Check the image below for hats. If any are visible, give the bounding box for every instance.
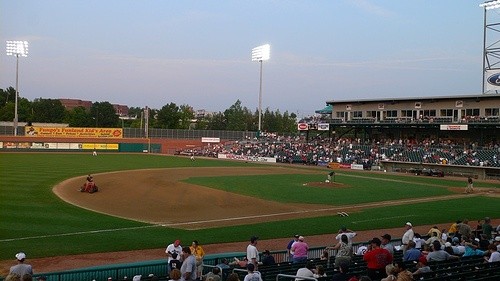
[294,234,300,238]
[16,252,26,260]
[250,236,258,241]
[298,236,304,241]
[452,237,459,242]
[406,222,412,227]
[371,238,381,246]
[263,249,270,252]
[484,217,489,221]
[381,234,391,240]
[419,256,427,264]
[174,240,180,246]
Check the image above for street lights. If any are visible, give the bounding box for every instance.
[249,41,272,130]
[4,38,30,126]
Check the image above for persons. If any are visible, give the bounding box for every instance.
[86,113,500,281]
[4,252,34,281]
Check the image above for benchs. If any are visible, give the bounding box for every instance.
[230,145,500,281]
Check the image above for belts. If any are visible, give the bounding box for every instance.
[402,244,408,245]
